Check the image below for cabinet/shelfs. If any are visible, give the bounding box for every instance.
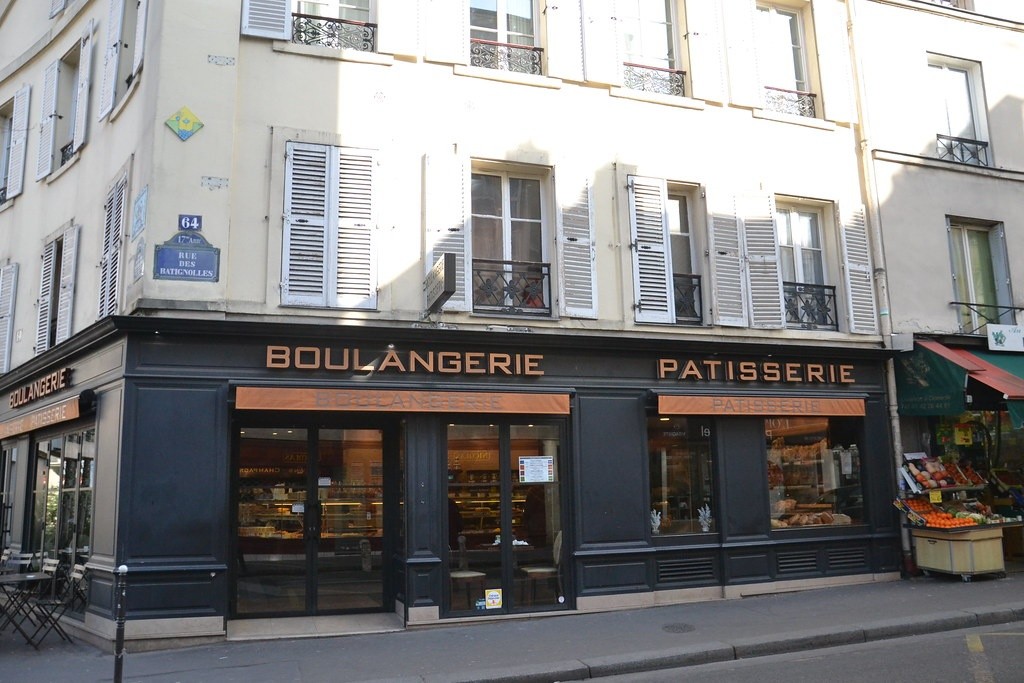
[770,459,825,489]
[239,483,546,553]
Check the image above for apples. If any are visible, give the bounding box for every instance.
[944,463,967,484]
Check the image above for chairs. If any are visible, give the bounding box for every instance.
[0,548,87,648]
[522,529,563,606]
[449,536,486,608]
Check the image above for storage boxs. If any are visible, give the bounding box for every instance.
[893,457,1024,526]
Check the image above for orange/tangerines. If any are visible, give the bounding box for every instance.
[902,499,977,528]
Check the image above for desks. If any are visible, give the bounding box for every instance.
[907,522,1024,581]
[0,572,53,651]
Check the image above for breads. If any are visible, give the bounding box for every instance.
[767,435,822,484]
[770,512,834,527]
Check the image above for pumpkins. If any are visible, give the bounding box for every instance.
[908,462,955,488]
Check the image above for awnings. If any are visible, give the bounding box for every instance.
[913,340,1024,399]
[896,350,966,417]
